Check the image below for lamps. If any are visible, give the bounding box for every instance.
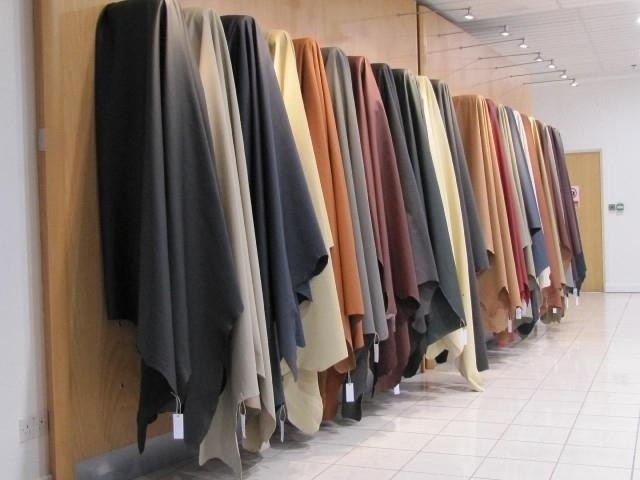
[478,51,545,62]
[460,37,528,49]
[398,6,475,20]
[438,24,510,36]
[495,58,555,69]
[523,78,580,87]
[510,69,568,79]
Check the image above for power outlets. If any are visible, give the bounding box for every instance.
[608,204,615,210]
